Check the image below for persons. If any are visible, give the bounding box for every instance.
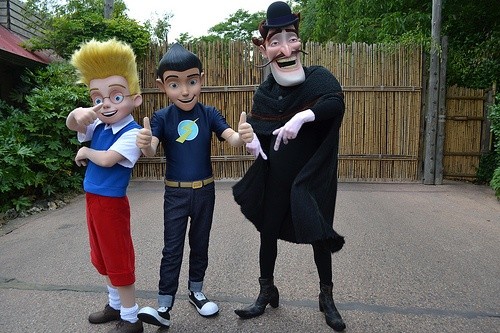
[136,43,253,328]
[66,39,142,333]
[232,1,347,331]
